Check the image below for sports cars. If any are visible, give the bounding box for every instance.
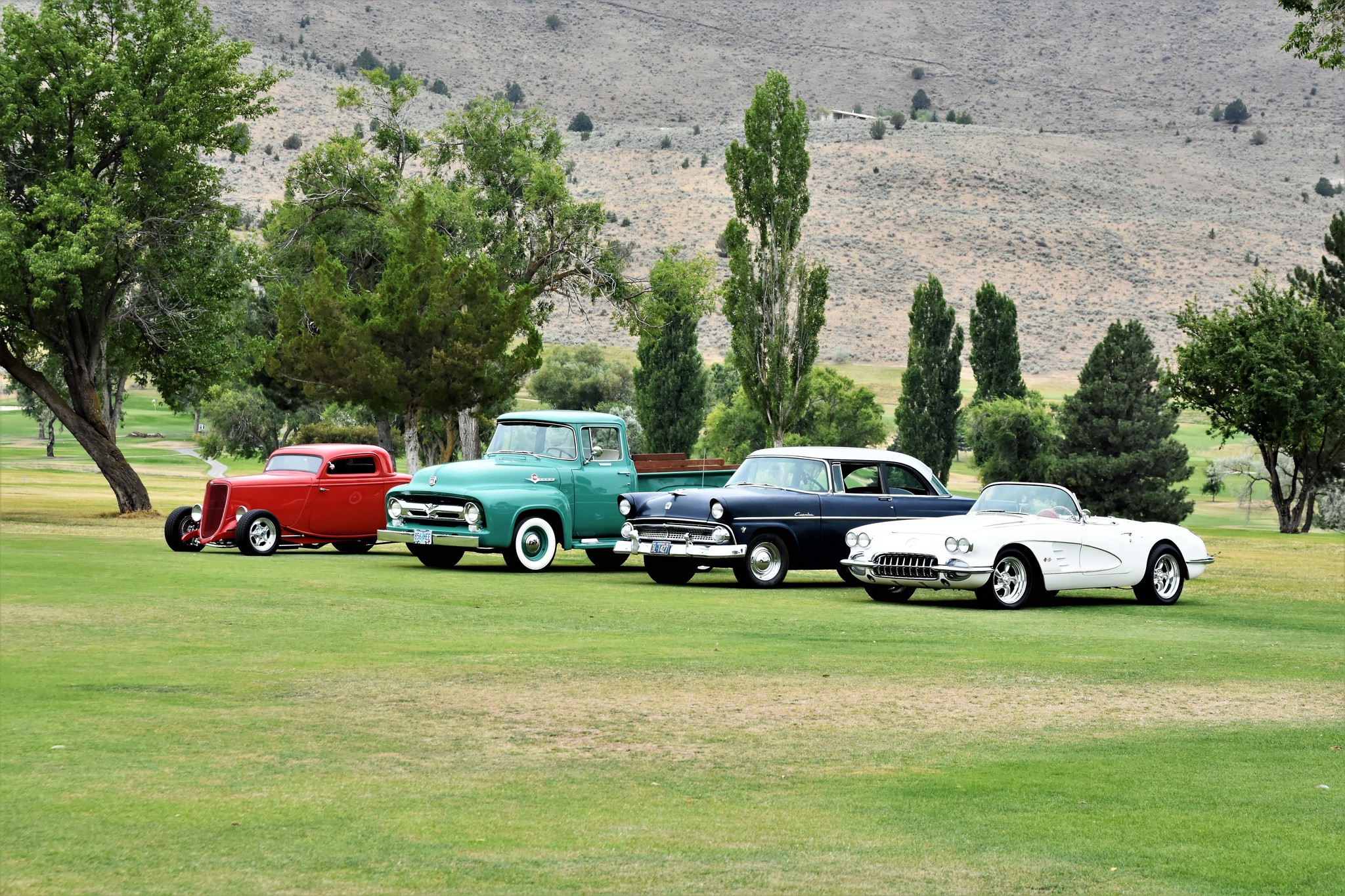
[840,480,1216,610]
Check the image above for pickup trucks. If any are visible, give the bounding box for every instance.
[377,409,741,573]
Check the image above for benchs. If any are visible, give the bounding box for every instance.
[335,463,374,472]
[545,445,619,461]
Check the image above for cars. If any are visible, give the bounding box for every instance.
[612,444,1023,591]
[163,442,409,556]
